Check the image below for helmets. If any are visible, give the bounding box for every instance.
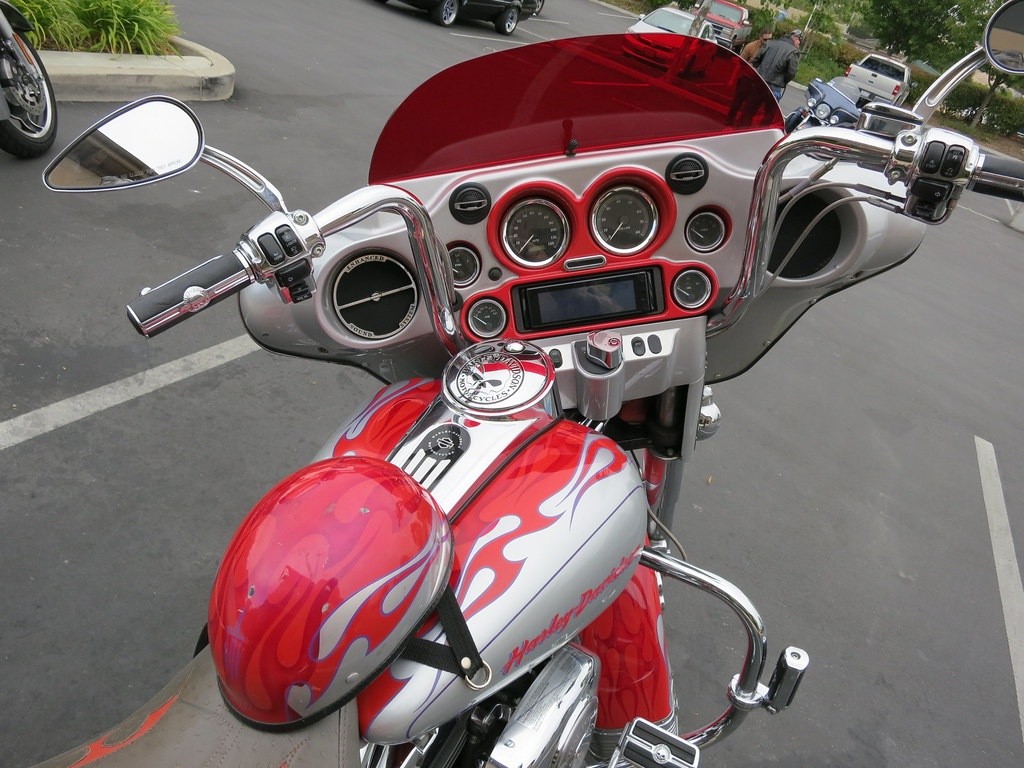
[208,455,454,734]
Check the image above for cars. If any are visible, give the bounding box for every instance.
[377,0,545,36]
[622,6,719,77]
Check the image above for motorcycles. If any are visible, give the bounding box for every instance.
[1,1,57,159]
[29,0,1023,767]
[785,76,862,134]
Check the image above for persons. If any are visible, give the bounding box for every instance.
[725,27,804,129]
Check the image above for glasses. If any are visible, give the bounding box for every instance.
[761,37,773,41]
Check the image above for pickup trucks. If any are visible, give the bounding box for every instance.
[842,53,918,108]
[691,0,753,55]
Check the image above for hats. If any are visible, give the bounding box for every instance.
[791,30,805,45]
